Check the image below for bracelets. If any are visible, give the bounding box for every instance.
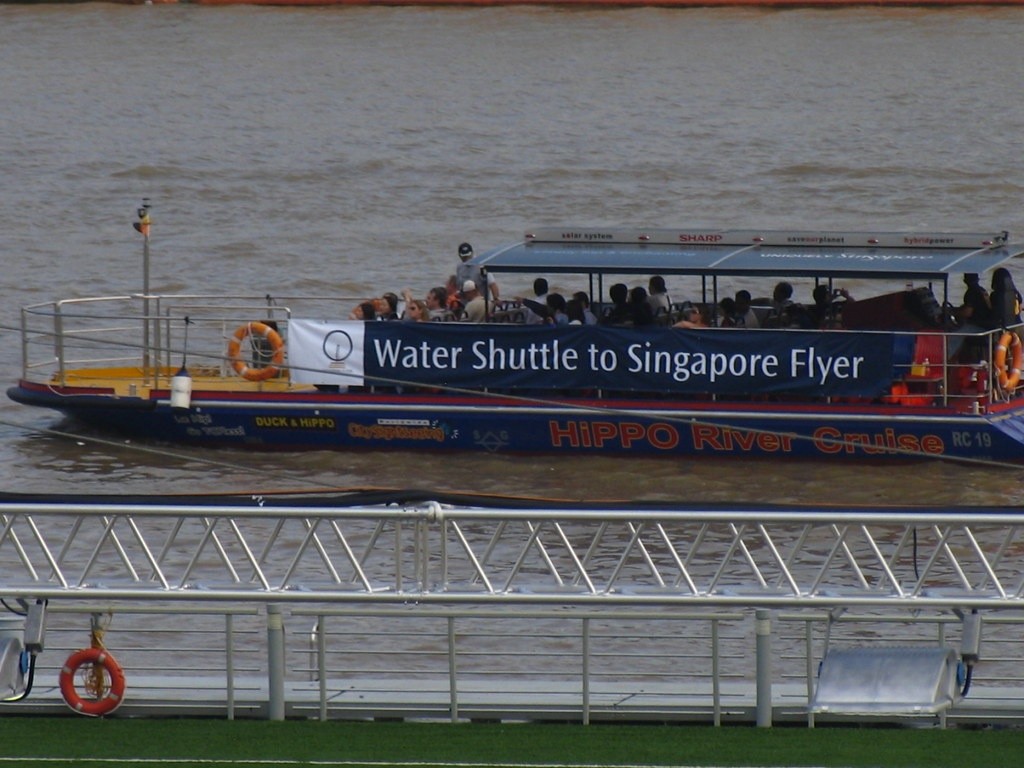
[493,296,500,301]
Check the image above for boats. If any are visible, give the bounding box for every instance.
[7,196,1024,474]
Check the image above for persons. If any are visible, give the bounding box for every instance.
[751,281,817,330]
[719,290,759,329]
[812,284,857,330]
[513,278,598,326]
[605,275,676,326]
[977,267,1023,330]
[673,303,709,328]
[949,273,992,321]
[353,243,503,325]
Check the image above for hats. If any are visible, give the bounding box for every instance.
[458,243,473,257]
[384,293,399,303]
[462,280,477,292]
[436,287,449,297]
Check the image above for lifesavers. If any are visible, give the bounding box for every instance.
[994,330,1023,394]
[59,648,125,715]
[227,321,284,381]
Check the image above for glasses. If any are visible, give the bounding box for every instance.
[408,307,416,311]
[690,310,698,314]
